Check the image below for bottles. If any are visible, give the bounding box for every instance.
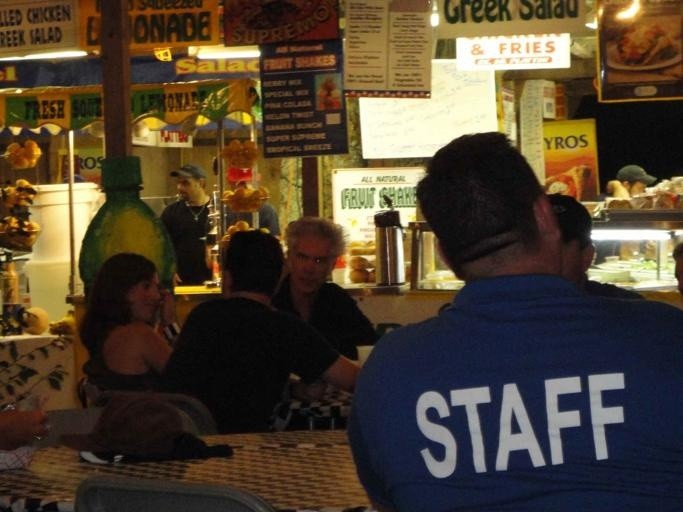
[407,218,438,290]
[74,151,179,334]
[1,250,20,305]
[372,207,406,289]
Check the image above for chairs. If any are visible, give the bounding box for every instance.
[69,374,217,435]
[73,474,277,512]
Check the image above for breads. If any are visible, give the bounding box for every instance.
[405,264,412,282]
[348,241,378,282]
[604,190,683,209]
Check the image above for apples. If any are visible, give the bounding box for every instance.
[316,80,341,110]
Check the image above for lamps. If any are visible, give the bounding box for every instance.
[0,49,95,62]
[187,44,260,60]
[584,0,598,29]
[430,0,440,27]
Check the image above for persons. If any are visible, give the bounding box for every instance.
[346,129,682,511]
[535,187,648,303]
[586,162,656,265]
[672,243,682,308]
[76,215,382,436]
[225,179,281,237]
[158,164,219,287]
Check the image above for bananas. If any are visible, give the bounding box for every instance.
[50,309,75,333]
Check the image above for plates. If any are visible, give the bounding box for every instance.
[605,34,683,72]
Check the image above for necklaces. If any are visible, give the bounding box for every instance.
[185,198,207,221]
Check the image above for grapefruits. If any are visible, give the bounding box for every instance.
[23,306,49,335]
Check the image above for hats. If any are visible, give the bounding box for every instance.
[616,165,657,185]
[169,164,206,177]
